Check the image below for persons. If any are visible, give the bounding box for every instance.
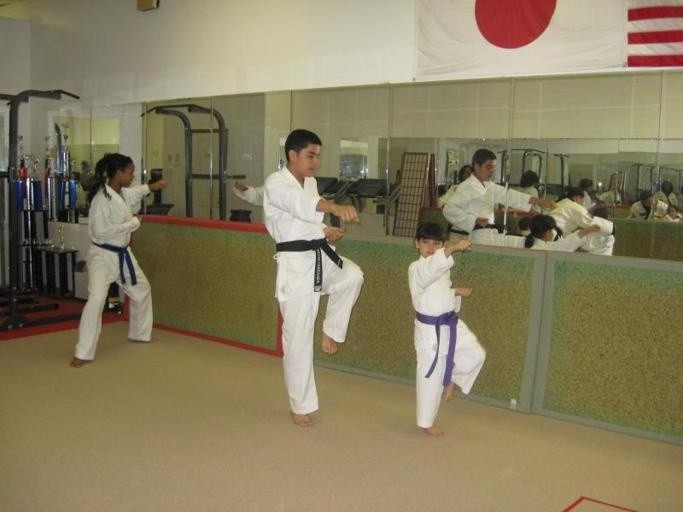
[263,129,364,427]
[437,148,683,255]
[71,154,168,368]
[228,177,265,207]
[408,223,486,437]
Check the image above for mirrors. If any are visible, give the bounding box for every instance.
[47,68,682,263]
